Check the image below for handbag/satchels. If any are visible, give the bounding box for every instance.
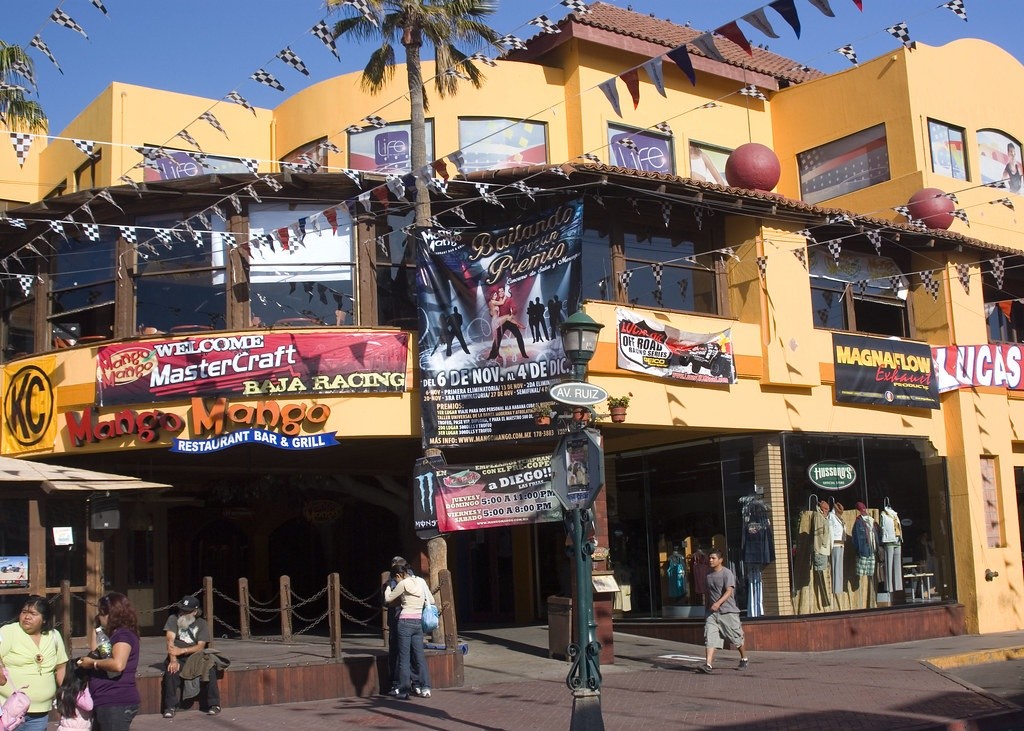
[0,691,31,731]
[422,586,438,632]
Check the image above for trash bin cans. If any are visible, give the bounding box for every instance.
[547,595,573,661]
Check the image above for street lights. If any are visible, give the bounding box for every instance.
[559,300,605,731]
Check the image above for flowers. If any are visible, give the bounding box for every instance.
[606,391,633,412]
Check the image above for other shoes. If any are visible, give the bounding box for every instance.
[208,705,221,715]
[163,706,175,717]
[413,686,431,698]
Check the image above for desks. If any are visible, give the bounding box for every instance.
[904,573,935,600]
[170,325,214,331]
[77,335,108,344]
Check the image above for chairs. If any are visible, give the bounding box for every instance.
[275,317,322,326]
[138,323,164,335]
[51,338,75,348]
[334,309,346,326]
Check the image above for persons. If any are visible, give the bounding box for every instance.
[56,592,142,731]
[697,548,749,675]
[0,595,69,731]
[1002,143,1024,194]
[385,556,435,700]
[527,294,566,343]
[487,286,529,360]
[431,306,470,356]
[666,549,710,605]
[161,596,222,718]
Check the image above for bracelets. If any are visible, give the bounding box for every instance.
[94,662,98,670]
[184,648,187,655]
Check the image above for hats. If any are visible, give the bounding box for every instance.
[820,501,830,516]
[856,501,867,515]
[175,595,200,611]
[835,501,844,521]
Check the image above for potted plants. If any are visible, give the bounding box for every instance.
[568,402,596,424]
[531,404,551,426]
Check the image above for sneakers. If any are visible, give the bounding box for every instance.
[395,692,410,699]
[697,664,715,675]
[387,688,399,696]
[737,658,748,670]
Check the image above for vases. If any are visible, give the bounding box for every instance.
[609,407,627,422]
